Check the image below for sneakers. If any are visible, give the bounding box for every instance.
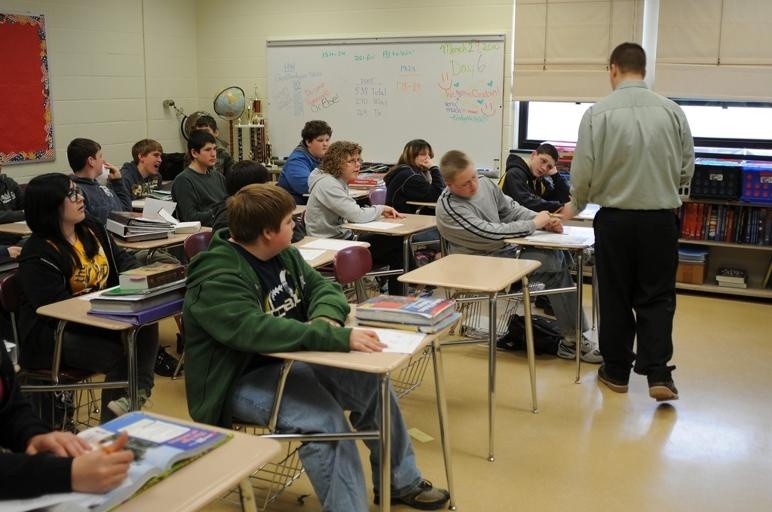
[103,387,153,419]
[372,478,451,510]
[646,374,681,404]
[155,345,184,379]
[594,363,631,395]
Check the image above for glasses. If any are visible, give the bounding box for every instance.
[345,158,364,165]
[64,186,82,202]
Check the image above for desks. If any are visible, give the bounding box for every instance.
[566,205,598,330]
[506,225,599,383]
[398,253,538,462]
[0,188,463,510]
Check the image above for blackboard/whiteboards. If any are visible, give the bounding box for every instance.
[266,34,506,178]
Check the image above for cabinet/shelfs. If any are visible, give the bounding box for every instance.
[675,195,772,302]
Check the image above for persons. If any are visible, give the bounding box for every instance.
[497,141,571,317]
[559,41,696,402]
[184,112,235,177]
[277,119,334,206]
[1,173,26,226]
[117,138,164,212]
[210,159,271,231]
[0,336,135,501]
[179,180,452,512]
[65,135,182,378]
[381,138,445,215]
[171,129,228,229]
[300,138,410,297]
[11,171,161,424]
[434,149,604,365]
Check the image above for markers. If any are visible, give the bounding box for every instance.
[477,168,490,171]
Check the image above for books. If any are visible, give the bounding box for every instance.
[352,293,462,334]
[716,267,748,289]
[348,176,386,190]
[148,181,174,201]
[103,206,202,243]
[673,201,772,249]
[761,259,772,289]
[42,407,233,510]
[85,261,189,327]
[678,247,712,264]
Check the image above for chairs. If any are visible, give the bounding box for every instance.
[440,222,505,335]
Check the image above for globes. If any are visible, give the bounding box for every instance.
[213,86,245,126]
[181,111,209,141]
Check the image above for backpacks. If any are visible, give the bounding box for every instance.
[555,332,607,364]
[494,311,567,358]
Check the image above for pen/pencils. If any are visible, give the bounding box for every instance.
[101,446,112,454]
